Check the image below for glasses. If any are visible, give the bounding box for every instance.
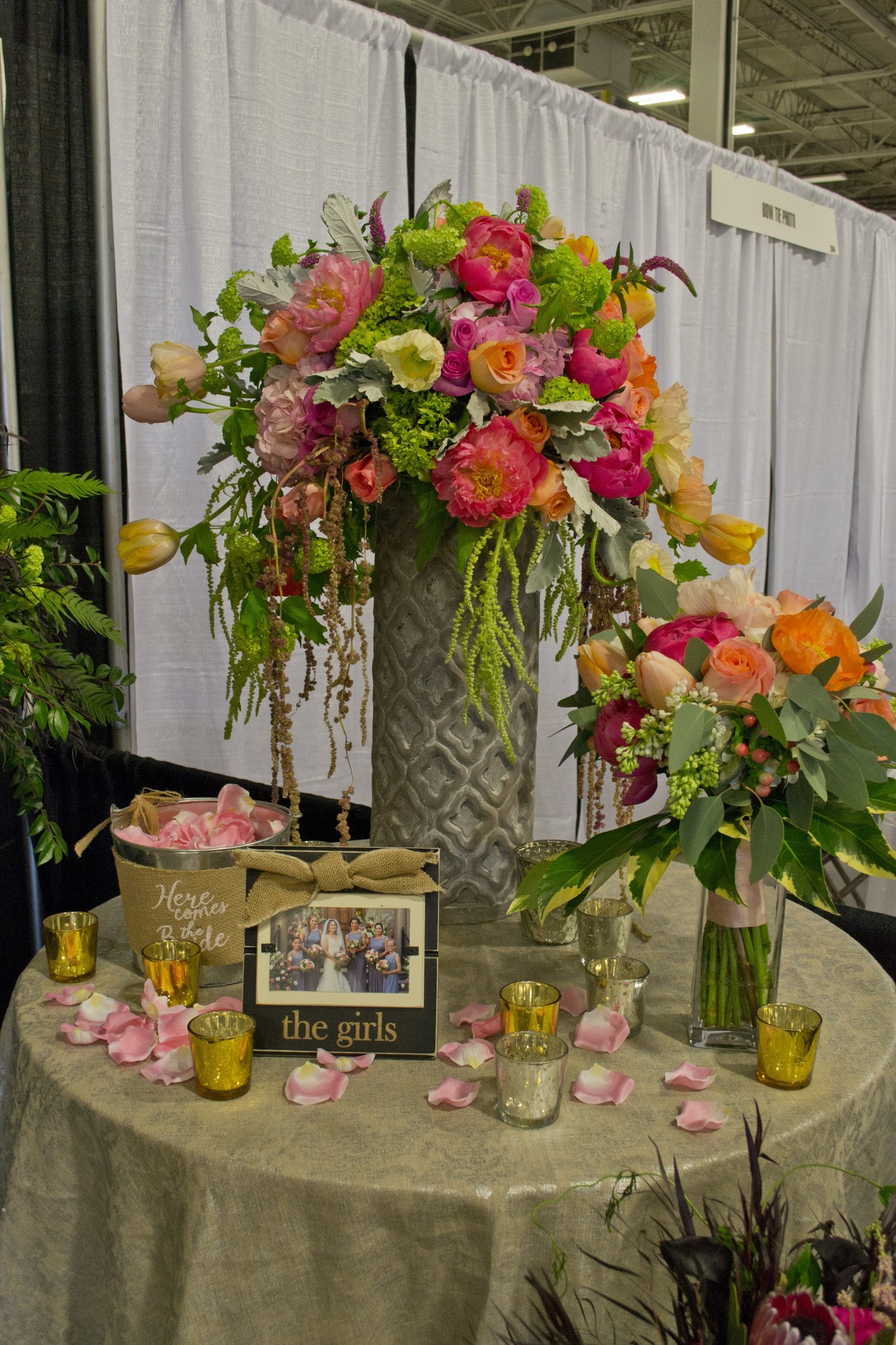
[387,945,395,947]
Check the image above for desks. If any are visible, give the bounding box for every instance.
[0,840,896,1345]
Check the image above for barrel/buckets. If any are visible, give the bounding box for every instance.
[110,797,294,987]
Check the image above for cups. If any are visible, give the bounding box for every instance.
[493,1031,569,1129]
[513,840,622,950]
[576,898,634,966]
[585,956,649,1040]
[499,980,562,1036]
[754,1002,823,1091]
[142,939,201,1008]
[187,1010,255,1101]
[42,911,99,983]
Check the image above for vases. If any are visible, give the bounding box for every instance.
[0,703,40,1028]
[689,820,786,1052]
[372,472,539,923]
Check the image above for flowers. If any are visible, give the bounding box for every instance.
[346,939,362,958]
[364,948,379,968]
[287,907,327,948]
[512,566,896,1029]
[356,908,394,948]
[308,944,322,960]
[375,959,389,978]
[300,958,315,978]
[0,467,135,867]
[400,955,409,988]
[118,179,765,846]
[334,951,351,970]
[269,948,298,991]
[461,1098,894,1345]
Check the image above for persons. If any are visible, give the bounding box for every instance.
[288,913,402,994]
